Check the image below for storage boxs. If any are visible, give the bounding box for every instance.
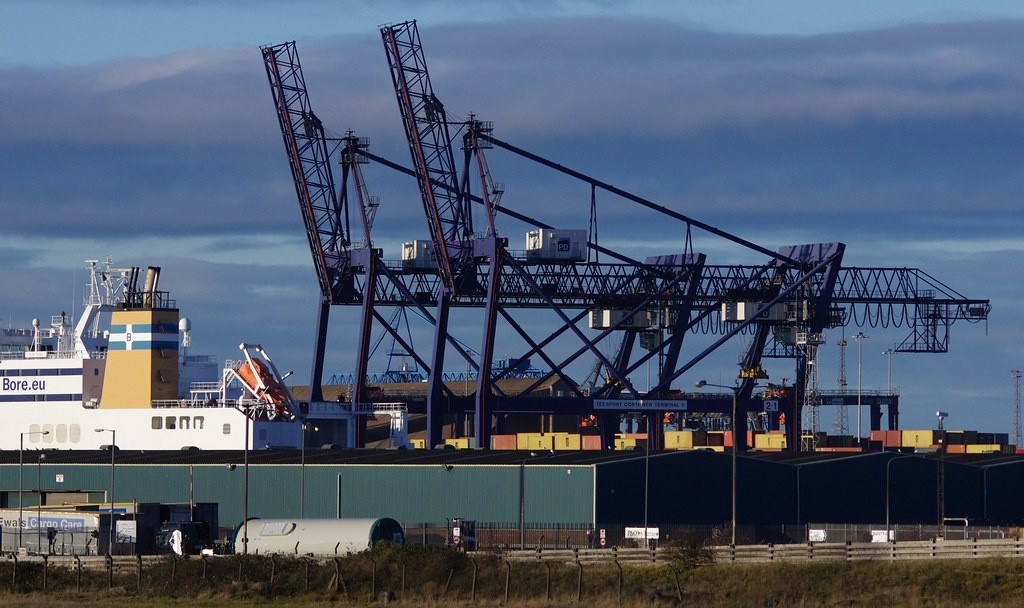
[868,428,1016,456]
[407,430,826,451]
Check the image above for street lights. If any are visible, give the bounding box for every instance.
[851,331,869,444]
[886,455,927,541]
[19,431,49,556]
[882,348,897,396]
[37,453,47,555]
[208,397,284,553]
[695,380,775,546]
[95,428,115,555]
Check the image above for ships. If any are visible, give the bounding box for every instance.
[0,254,309,450]
